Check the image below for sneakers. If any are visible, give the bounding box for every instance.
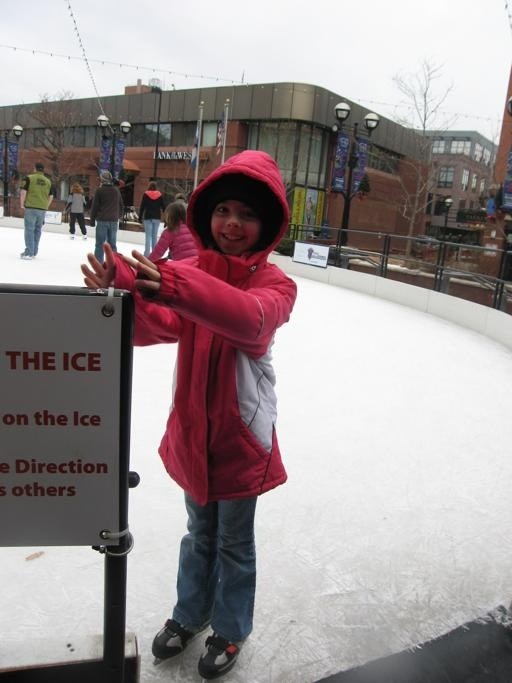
[70,233,87,239]
[198,633,244,679]
[152,618,212,659]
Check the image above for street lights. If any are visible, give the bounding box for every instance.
[443,198,453,241]
[332,103,381,246]
[149,85,161,191]
[4,124,23,217]
[97,115,131,179]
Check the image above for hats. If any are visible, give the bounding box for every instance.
[99,171,112,181]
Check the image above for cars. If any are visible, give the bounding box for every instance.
[413,234,442,258]
[449,249,480,272]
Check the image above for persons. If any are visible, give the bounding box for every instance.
[173,192,190,208]
[137,180,166,257]
[82,149,299,676]
[20,161,55,260]
[88,171,125,263]
[62,183,88,238]
[147,201,197,262]
